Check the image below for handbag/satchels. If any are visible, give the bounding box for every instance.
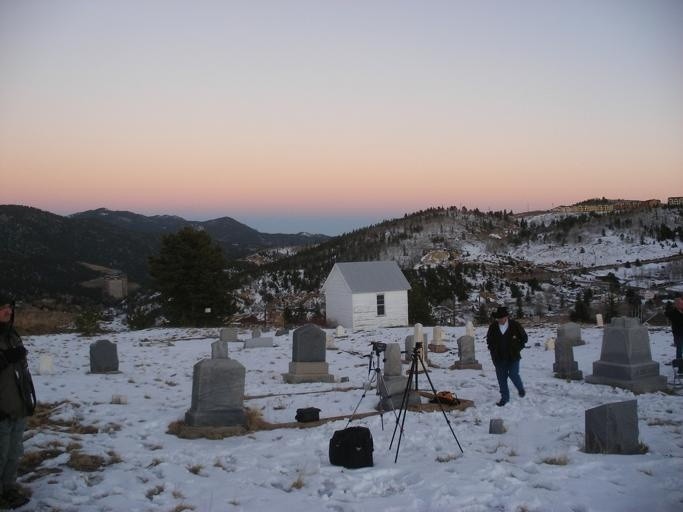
[327,425,376,469]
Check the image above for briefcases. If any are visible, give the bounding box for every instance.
[293,407,322,422]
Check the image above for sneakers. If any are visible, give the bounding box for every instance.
[495,397,509,406]
[519,389,525,397]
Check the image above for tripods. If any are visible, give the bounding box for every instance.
[344,354,405,436]
[389,353,464,463]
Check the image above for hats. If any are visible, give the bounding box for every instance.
[491,307,510,319]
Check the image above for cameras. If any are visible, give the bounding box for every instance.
[369,340,387,353]
[414,341,424,353]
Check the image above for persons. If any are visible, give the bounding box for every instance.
[664,293,683,374]
[0,294,36,509]
[487,307,528,406]
